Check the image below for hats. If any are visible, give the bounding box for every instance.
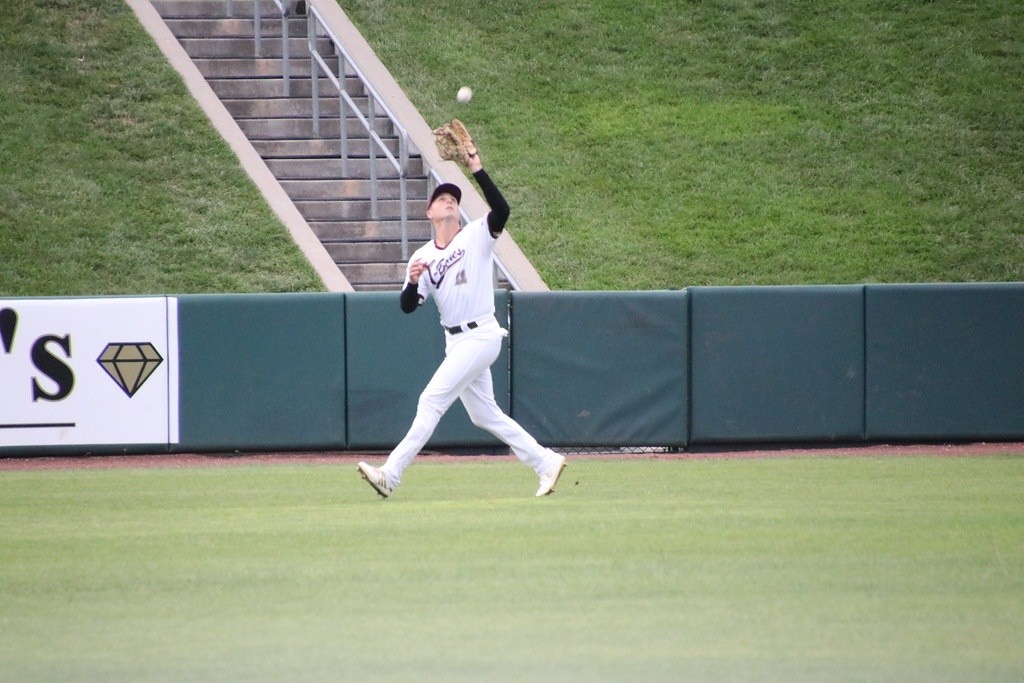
[428,184,462,210]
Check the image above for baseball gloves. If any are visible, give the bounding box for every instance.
[431,118,481,167]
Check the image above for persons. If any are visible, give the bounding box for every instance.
[356,119,568,498]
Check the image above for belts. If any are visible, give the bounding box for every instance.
[447,322,478,336]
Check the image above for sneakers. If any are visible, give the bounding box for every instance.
[535,448,567,497]
[356,461,393,499]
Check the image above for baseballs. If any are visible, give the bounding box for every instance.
[456,86,473,104]
